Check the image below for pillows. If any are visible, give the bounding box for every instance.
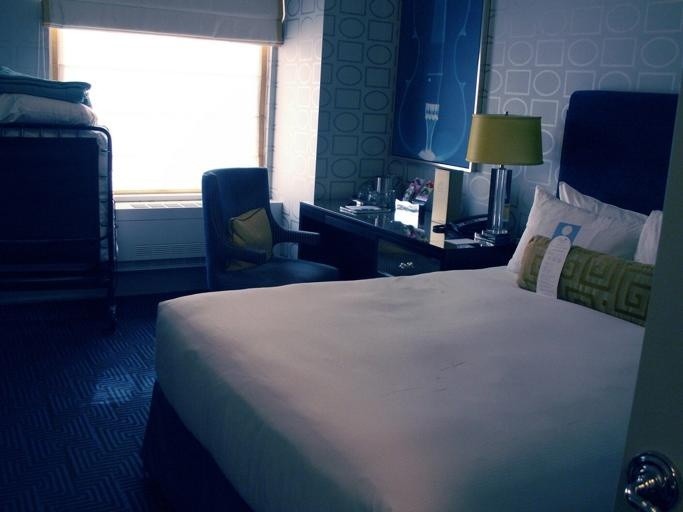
[0,89,101,129]
[222,206,274,273]
[557,179,647,226]
[632,209,663,266]
[516,235,656,326]
[505,185,641,276]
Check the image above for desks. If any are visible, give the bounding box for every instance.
[298,199,512,281]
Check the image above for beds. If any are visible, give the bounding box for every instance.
[0,121,119,306]
[138,90,681,511]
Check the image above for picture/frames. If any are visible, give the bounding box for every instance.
[384,0,497,176]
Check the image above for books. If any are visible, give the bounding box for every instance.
[338,200,391,218]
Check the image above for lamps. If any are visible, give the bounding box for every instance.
[464,110,544,245]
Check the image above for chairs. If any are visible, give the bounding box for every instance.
[200,166,343,293]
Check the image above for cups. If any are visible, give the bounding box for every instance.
[357,187,399,205]
[365,208,400,229]
[376,177,393,195]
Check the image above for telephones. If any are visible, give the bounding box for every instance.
[450,213,492,234]
